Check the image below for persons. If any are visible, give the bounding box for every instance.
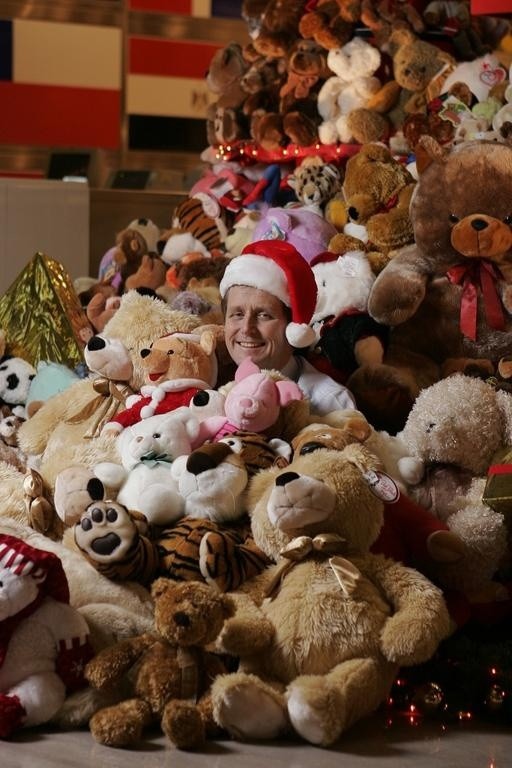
[219,239,357,418]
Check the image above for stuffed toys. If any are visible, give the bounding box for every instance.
[0,0,512,753]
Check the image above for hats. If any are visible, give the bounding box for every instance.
[223,241,322,349]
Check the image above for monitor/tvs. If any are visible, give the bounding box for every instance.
[108,169,151,191]
[47,151,90,176]
